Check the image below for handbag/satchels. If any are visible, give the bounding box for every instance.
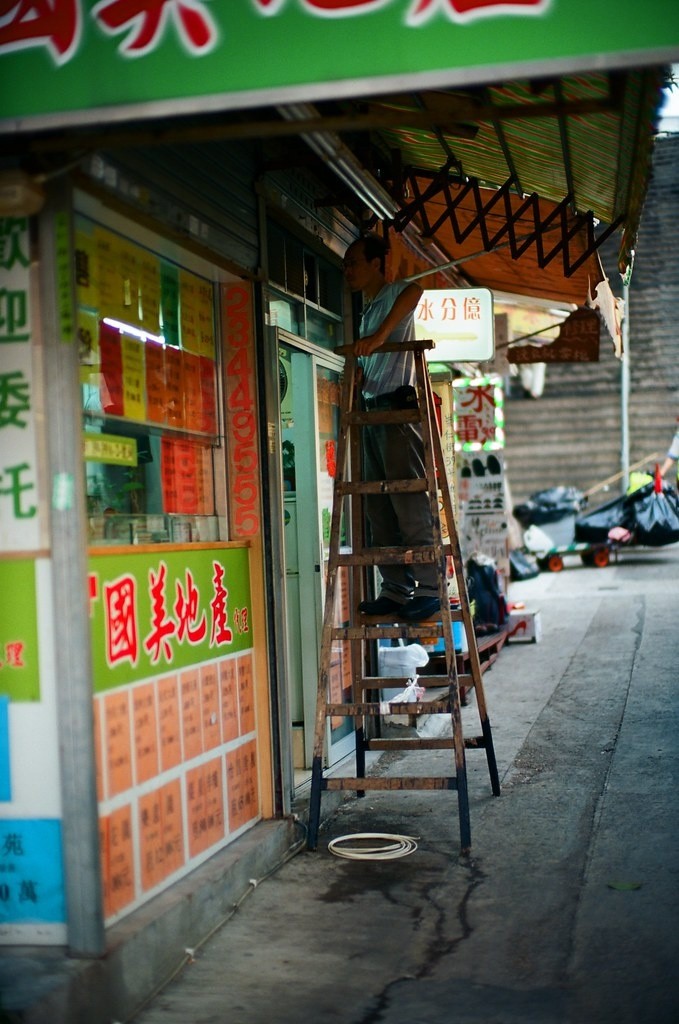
[394,385,418,409]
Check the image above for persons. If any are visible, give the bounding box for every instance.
[660,415,679,492]
[338,235,447,621]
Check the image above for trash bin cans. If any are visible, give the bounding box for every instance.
[524,488,577,552]
[379,646,420,712]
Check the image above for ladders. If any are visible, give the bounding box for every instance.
[305,338,505,864]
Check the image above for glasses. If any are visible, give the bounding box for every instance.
[342,257,372,269]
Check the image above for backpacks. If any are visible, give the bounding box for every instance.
[465,552,510,630]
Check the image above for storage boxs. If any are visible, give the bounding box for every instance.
[539,515,575,545]
[394,597,462,655]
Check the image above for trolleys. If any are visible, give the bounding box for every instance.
[525,471,662,573]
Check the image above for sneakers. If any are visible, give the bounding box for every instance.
[358,597,404,616]
[399,596,440,620]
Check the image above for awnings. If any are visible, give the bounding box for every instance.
[0,0,679,362]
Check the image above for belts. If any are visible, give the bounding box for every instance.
[366,397,393,409]
